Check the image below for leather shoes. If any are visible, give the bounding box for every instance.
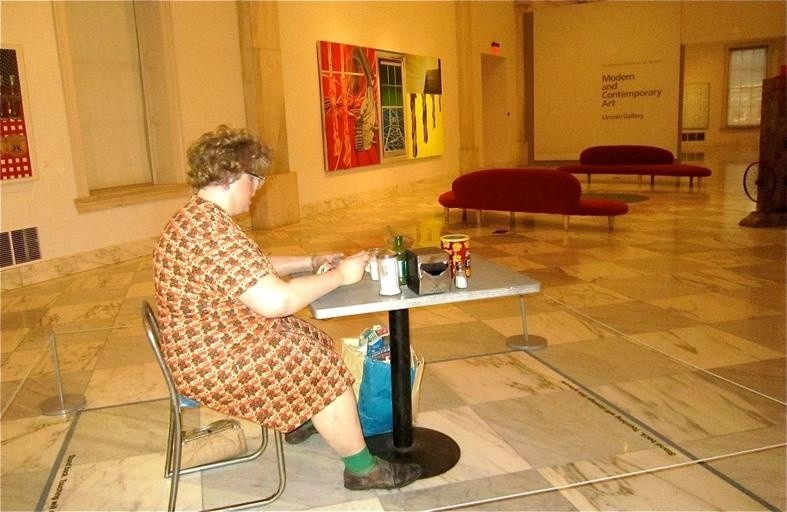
[285,421,320,444]
[343,457,422,491]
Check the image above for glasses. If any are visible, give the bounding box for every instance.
[244,169,267,190]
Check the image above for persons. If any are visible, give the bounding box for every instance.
[152,126,421,491]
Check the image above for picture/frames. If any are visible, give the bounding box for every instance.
[1,49,35,185]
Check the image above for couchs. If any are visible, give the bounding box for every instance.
[438,166,628,230]
[555,145,711,188]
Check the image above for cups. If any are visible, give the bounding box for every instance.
[365,248,384,281]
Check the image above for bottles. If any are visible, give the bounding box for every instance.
[391,235,409,285]
[378,251,400,295]
[455,261,467,288]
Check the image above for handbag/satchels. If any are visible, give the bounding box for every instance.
[338,338,426,439]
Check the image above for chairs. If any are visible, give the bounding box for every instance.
[138,300,286,512]
